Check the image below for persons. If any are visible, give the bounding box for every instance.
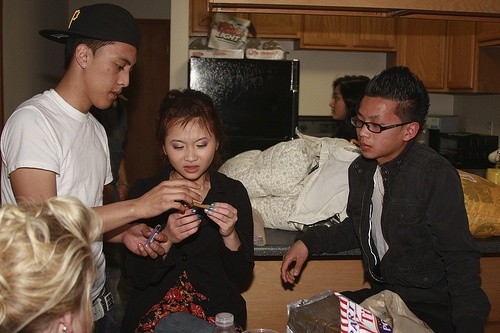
[0,3,202,333]
[0,195,103,333]
[113,88,255,333]
[328,75,371,144]
[279,65,492,333]
[88,93,134,333]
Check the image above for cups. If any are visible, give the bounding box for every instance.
[241,328,279,333]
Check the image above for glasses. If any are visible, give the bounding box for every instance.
[351,115,412,133]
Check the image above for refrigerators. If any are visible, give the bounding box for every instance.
[189,57,300,167]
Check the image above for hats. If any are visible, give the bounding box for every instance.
[38,2,140,46]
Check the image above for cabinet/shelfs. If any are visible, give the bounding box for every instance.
[188,0,500,94]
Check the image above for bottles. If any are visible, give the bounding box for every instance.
[416,118,429,145]
[211,313,238,333]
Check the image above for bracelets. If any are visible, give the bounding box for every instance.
[115,180,130,187]
